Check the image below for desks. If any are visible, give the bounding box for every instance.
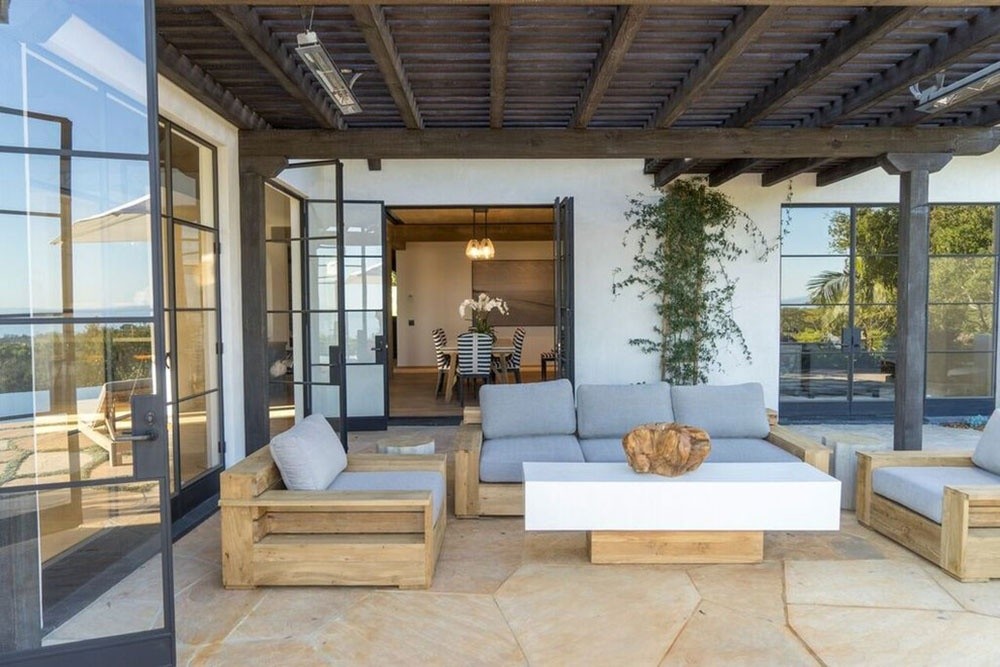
[439,338,516,402]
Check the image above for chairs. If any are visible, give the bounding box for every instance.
[494,327,525,384]
[432,327,451,399]
[79,378,151,466]
[541,352,554,381]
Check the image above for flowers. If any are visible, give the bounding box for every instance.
[459,293,509,338]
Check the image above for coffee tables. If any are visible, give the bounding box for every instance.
[522,461,842,565]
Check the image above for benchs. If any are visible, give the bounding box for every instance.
[455,332,493,408]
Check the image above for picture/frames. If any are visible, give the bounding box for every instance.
[471,259,556,327]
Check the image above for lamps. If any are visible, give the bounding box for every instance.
[910,60,1000,114]
[295,6,365,117]
[466,207,495,260]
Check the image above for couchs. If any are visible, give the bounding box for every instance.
[854,410,1000,583]
[218,412,448,593]
[455,377,833,520]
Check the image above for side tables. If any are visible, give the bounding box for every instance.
[376,433,436,458]
[822,431,887,512]
